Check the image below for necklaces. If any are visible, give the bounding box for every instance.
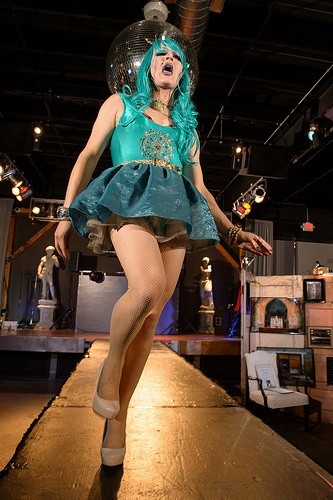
[148,99,169,116]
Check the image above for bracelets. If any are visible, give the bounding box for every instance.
[224,224,242,248]
[56,206,73,221]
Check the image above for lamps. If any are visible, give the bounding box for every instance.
[233,174,267,220]
[107,0,200,100]
[89,271,105,284]
[32,204,46,214]
[307,119,323,141]
[0,154,32,201]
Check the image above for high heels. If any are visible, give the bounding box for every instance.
[100,418,126,466]
[91,358,121,420]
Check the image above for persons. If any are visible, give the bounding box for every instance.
[226,303,239,338]
[37,245,59,301]
[199,257,215,310]
[54,36,273,468]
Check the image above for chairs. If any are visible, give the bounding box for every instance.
[246,351,308,429]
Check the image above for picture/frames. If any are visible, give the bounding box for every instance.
[302,279,326,303]
[306,326,332,349]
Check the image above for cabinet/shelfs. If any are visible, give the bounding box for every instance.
[249,274,315,389]
